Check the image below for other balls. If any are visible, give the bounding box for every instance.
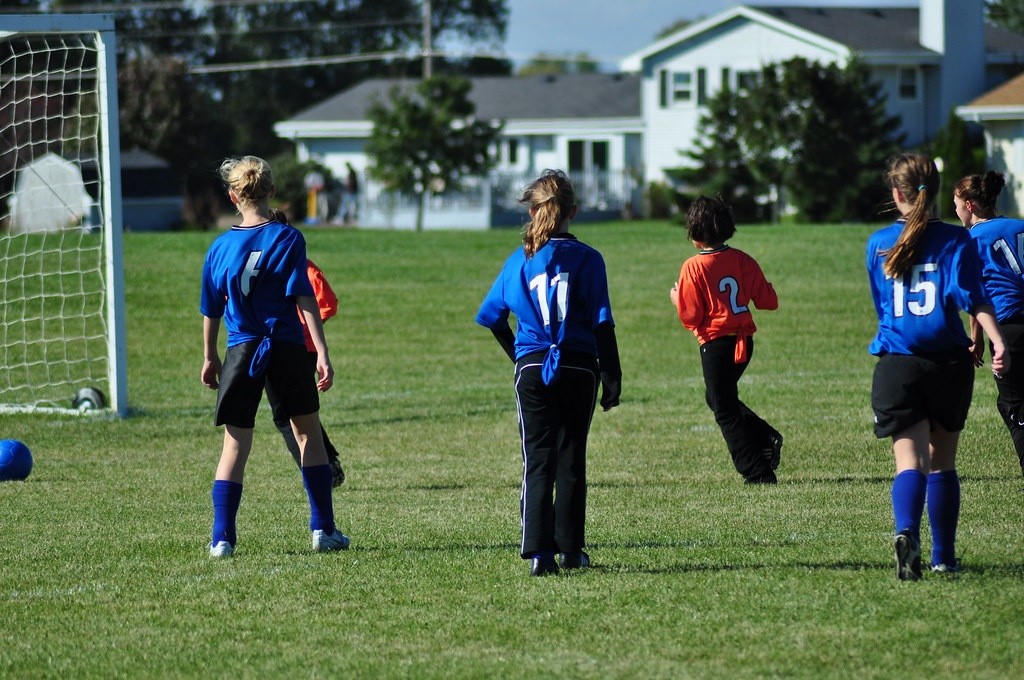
[72,386,107,409]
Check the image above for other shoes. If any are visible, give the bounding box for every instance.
[330,461,344,488]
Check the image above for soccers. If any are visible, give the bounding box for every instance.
[0,439,33,480]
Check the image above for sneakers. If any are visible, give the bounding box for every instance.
[312,528,350,552]
[205,540,233,556]
[559,551,589,568]
[893,530,923,581]
[931,558,961,573]
[531,556,559,576]
[763,429,783,470]
[744,472,777,484]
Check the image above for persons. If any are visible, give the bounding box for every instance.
[476,173,624,575]
[868,153,1024,583]
[199,156,351,557]
[670,193,785,485]
[304,163,358,226]
